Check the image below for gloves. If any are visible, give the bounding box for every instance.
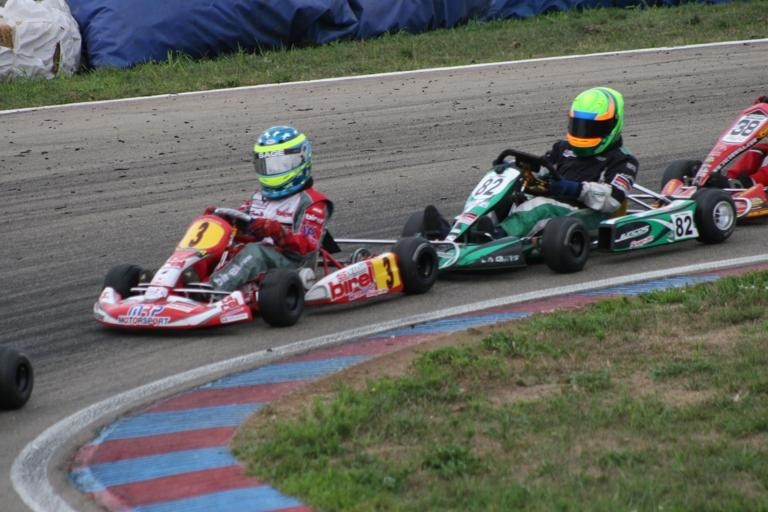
[549,176,579,199]
[247,218,281,239]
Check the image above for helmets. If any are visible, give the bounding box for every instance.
[566,87,625,157]
[253,126,312,200]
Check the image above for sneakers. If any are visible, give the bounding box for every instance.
[422,204,447,240]
[183,268,206,302]
[479,216,494,242]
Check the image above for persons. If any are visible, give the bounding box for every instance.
[422,86,640,239]
[688,138,768,189]
[139,125,334,301]
